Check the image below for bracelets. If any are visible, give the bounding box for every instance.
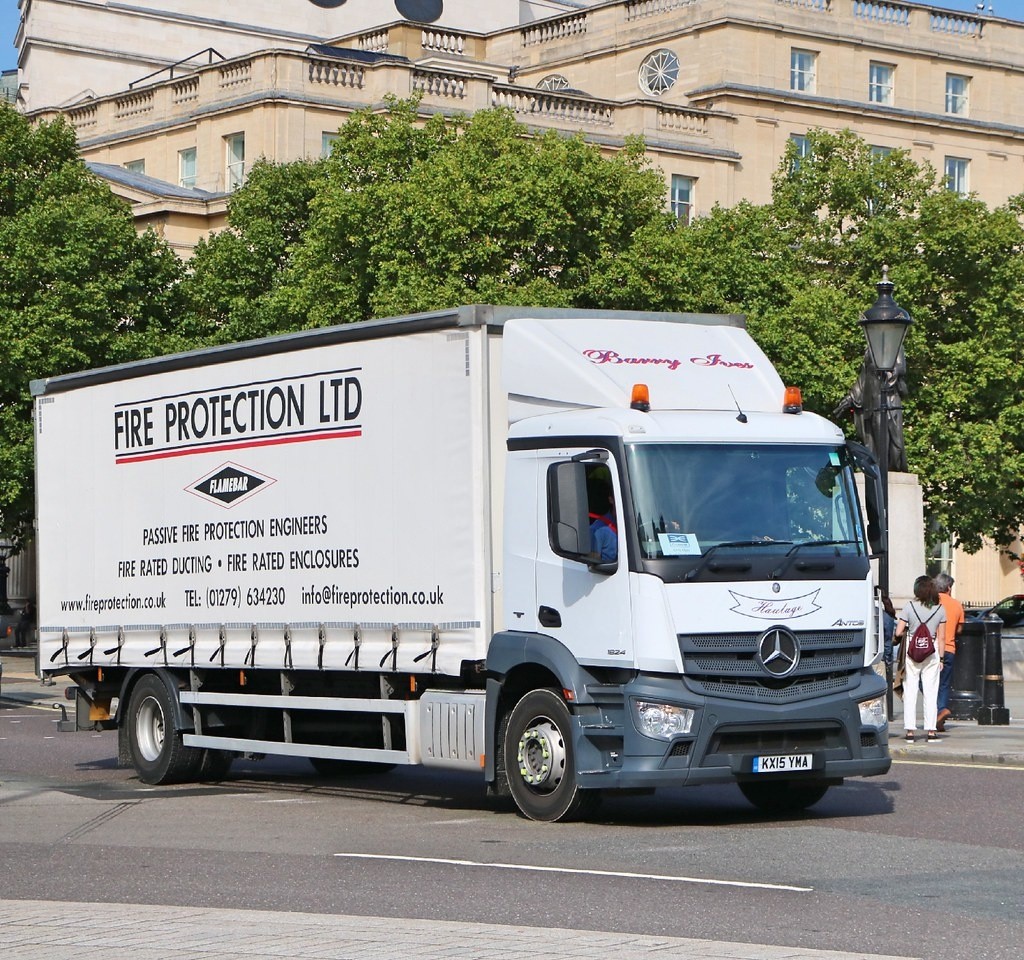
[940,657,945,663]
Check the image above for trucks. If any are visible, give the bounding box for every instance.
[26,304,894,822]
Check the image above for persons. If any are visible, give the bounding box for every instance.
[933,573,965,732]
[589,472,684,562]
[874,597,898,724]
[896,576,946,743]
[12,601,36,647]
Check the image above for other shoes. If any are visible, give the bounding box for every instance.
[889,718,894,723]
[937,709,951,732]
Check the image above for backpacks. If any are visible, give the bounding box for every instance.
[907,601,942,663]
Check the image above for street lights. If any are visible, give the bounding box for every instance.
[857,263,916,608]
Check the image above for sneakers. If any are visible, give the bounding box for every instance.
[904,734,915,744]
[927,734,943,743]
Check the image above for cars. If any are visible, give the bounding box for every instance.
[892,594,1023,681]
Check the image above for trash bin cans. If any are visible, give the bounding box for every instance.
[947,620,986,720]
